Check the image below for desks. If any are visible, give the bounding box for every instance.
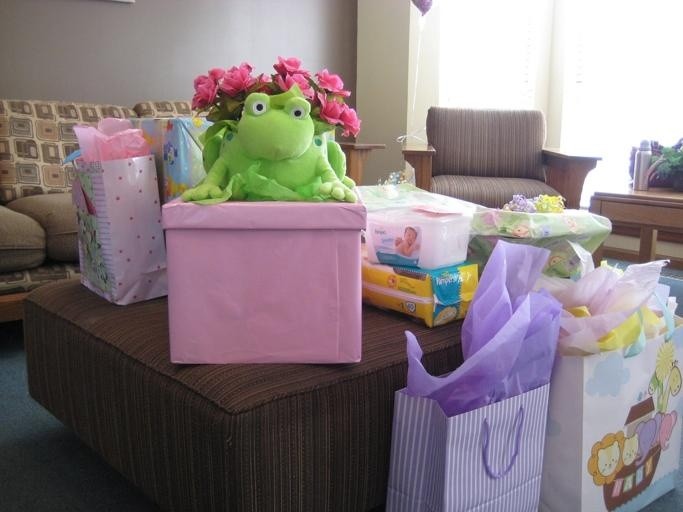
[589,186,683,274]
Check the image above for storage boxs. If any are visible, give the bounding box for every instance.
[161,196,363,365]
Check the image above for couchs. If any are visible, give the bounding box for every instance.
[22,242,606,512]
[1,97,387,328]
[403,105,602,211]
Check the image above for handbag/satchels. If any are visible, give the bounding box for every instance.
[384,377,550,512]
[540,316,683,511]
[65,152,167,306]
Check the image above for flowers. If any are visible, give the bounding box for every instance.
[501,195,567,214]
[188,57,364,141]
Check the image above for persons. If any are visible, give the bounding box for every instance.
[395,227,420,256]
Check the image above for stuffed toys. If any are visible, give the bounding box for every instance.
[179,92,360,207]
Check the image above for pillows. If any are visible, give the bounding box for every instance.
[0,204,47,274]
[12,193,81,265]
